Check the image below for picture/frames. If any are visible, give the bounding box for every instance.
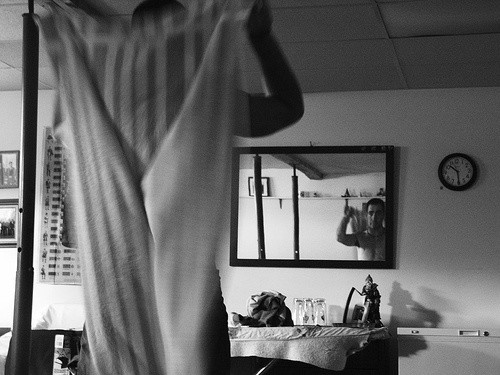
[248,175,270,198]
[0,150,20,189]
[0,199,20,248]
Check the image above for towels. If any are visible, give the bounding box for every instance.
[351,207,369,252]
[34,1,254,375]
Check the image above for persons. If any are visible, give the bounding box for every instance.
[0,218,16,238]
[336,198,386,262]
[49,0,306,375]
[0,162,16,186]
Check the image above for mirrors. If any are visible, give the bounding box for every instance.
[229,142,398,270]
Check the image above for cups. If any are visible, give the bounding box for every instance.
[294,297,305,325]
[315,298,327,327]
[303,298,315,325]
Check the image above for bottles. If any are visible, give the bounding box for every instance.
[377,187,385,196]
[344,188,350,196]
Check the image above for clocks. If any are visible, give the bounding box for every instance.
[437,153,478,191]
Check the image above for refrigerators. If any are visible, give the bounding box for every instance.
[395,328,500,375]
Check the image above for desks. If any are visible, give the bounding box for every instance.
[31,327,84,375]
[227,324,391,375]
